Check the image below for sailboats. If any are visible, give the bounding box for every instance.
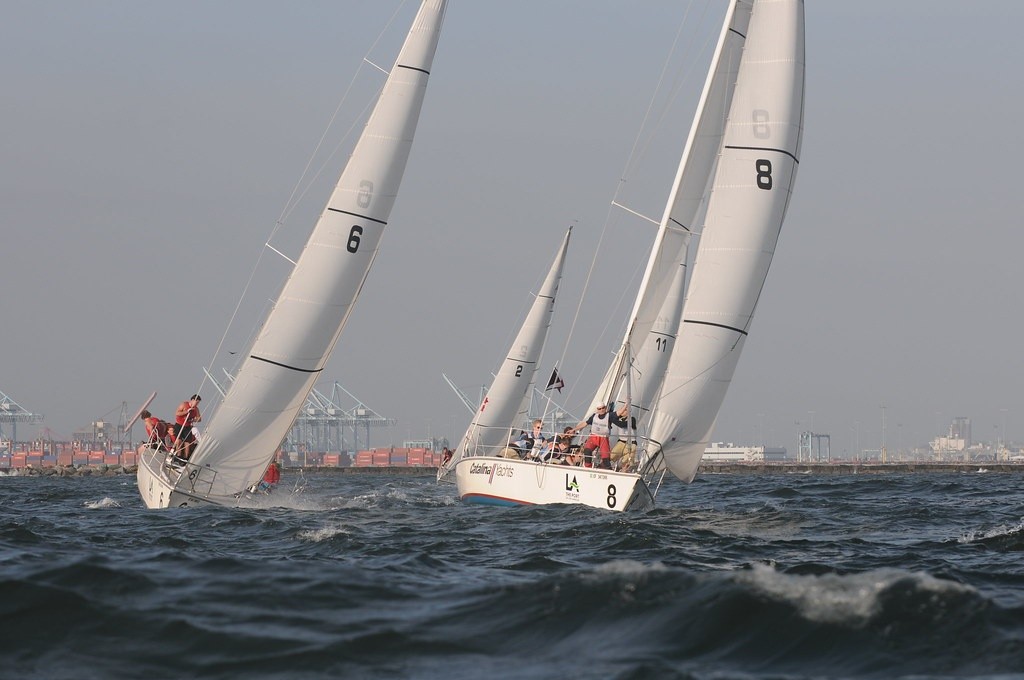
[456,0,806,516]
[137,42,440,511]
[437,225,575,487]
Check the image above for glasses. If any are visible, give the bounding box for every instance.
[537,427,542,429]
[597,406,605,410]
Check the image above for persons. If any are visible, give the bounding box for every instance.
[442,447,455,466]
[248,460,280,493]
[610,406,637,472]
[138,394,201,466]
[500,420,583,467]
[567,395,632,469]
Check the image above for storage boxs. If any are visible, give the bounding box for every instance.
[324,453,341,466]
[0,448,138,466]
[357,448,442,467]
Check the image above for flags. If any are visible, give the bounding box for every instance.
[546,368,565,394]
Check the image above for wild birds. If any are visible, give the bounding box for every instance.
[228,351,238,354]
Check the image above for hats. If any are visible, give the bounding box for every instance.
[596,403,605,408]
